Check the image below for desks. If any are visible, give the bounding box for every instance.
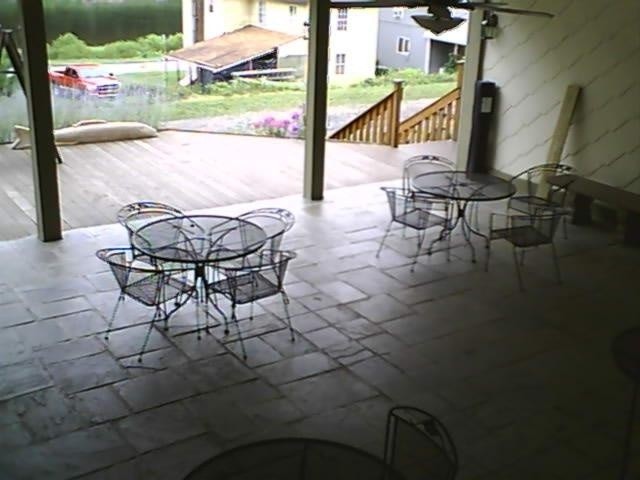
[183,437,409,480]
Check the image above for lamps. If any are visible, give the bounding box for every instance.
[481,14,497,40]
[410,6,466,35]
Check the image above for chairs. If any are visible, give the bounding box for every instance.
[383,403,460,479]
[374,155,580,292]
[95,200,298,363]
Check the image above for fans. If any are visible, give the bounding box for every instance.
[328,0,558,25]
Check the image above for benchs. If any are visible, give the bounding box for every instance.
[543,173,640,247]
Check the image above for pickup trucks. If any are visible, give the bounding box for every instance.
[49,62,121,102]
